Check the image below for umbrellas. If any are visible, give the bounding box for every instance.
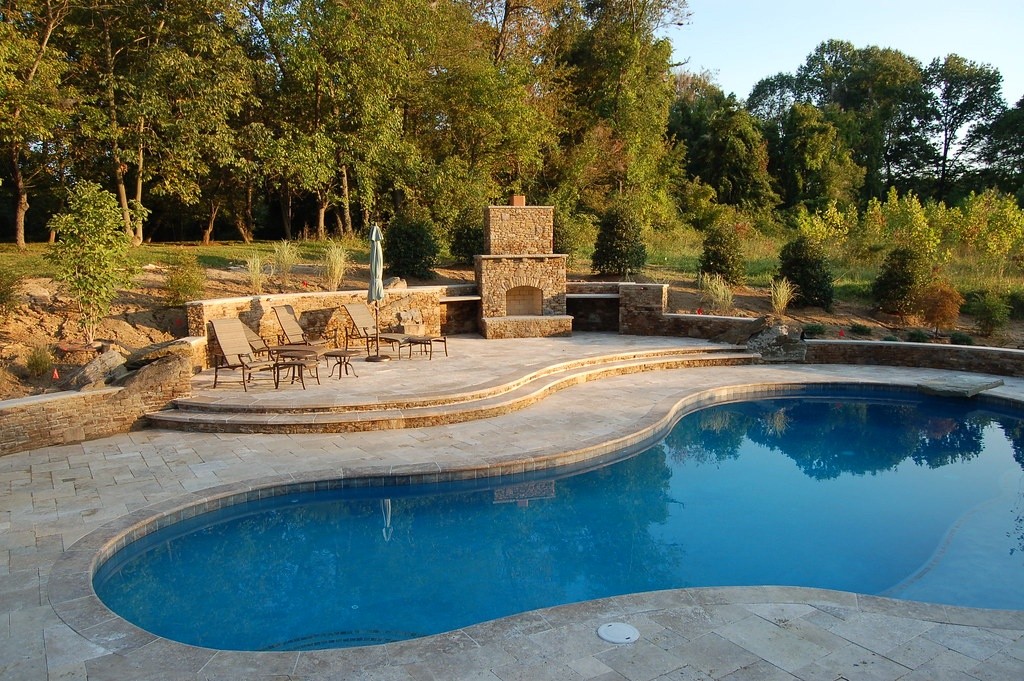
[366,221,392,363]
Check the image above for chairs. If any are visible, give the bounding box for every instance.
[342,303,412,361]
[208,305,338,391]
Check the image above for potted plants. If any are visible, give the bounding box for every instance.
[497,178,535,206]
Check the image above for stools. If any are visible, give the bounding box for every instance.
[272,360,320,390]
[409,335,448,360]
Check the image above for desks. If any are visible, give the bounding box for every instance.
[325,350,361,380]
[280,352,318,385]
